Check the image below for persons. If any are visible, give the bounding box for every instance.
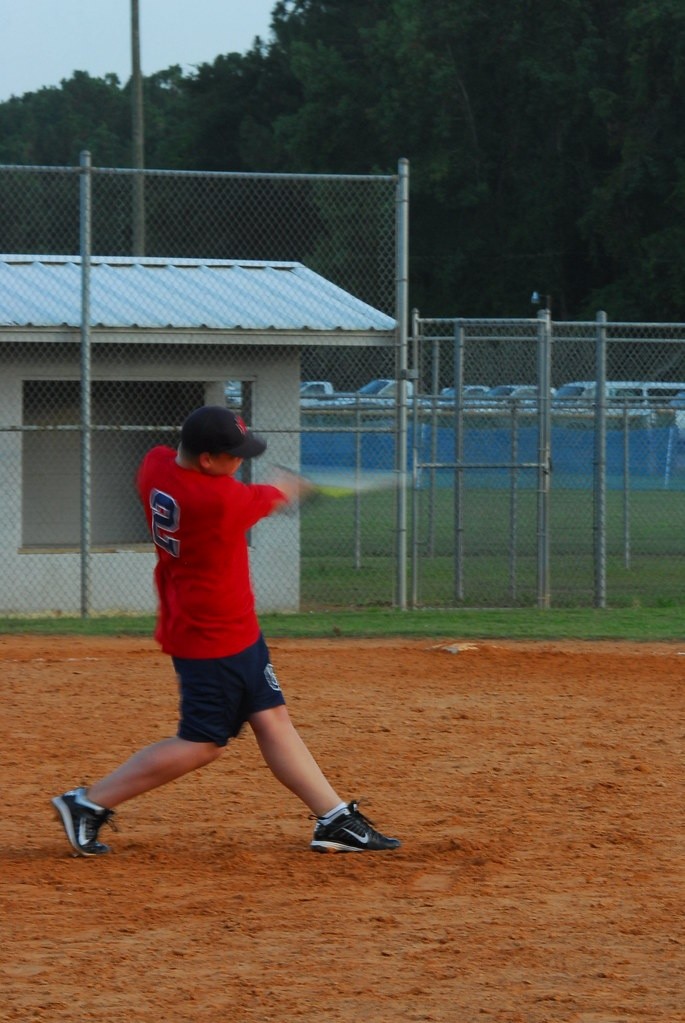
[48,409,404,856]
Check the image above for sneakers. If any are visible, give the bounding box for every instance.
[310,802,400,852]
[52,787,112,857]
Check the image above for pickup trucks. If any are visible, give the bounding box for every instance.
[298,381,353,405]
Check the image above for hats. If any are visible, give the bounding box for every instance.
[181,405,267,458]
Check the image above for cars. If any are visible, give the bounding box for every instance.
[426,386,489,409]
[465,384,556,413]
[337,380,413,405]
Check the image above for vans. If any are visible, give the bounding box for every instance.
[553,382,685,413]
